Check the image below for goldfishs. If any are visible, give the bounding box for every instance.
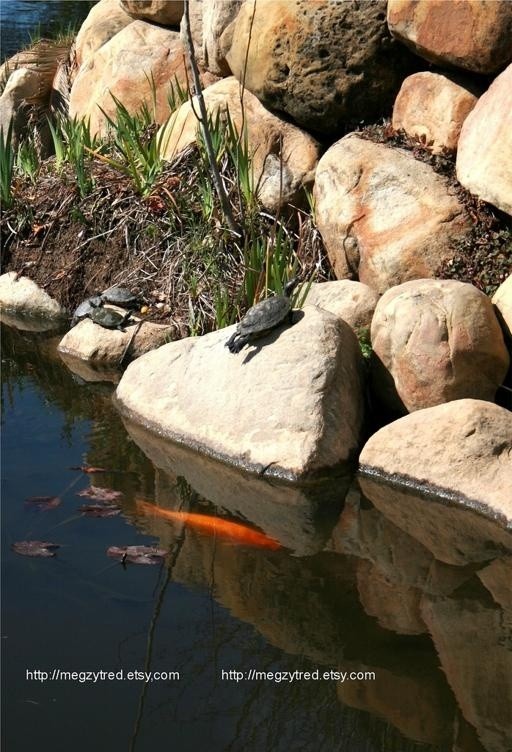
[134,498,282,552]
